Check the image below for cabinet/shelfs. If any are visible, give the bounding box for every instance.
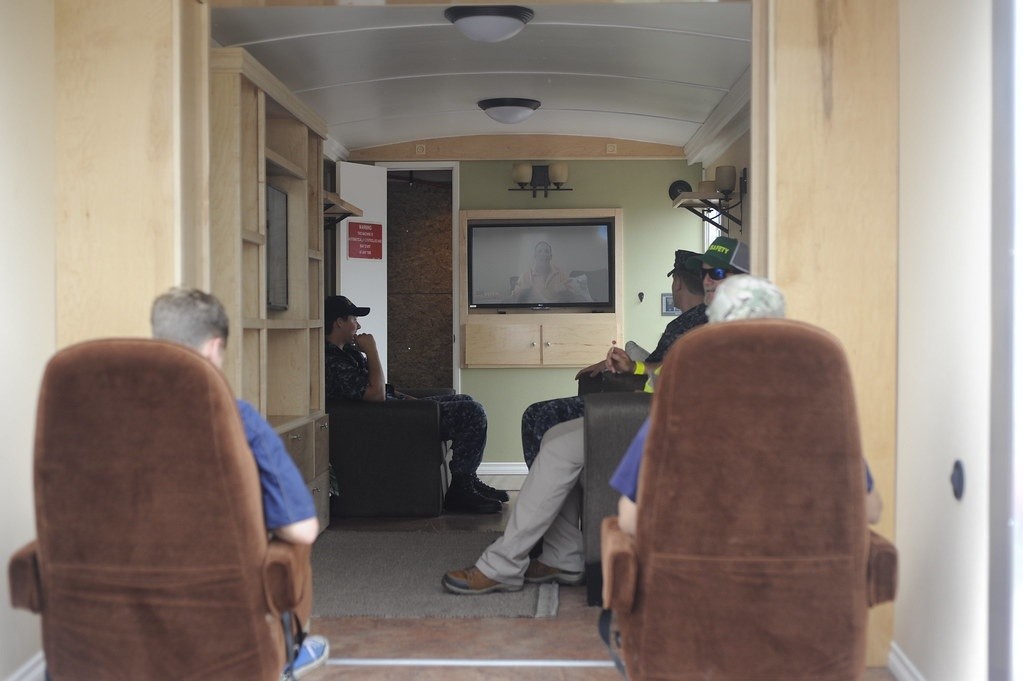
[207,47,331,548]
[459,208,625,369]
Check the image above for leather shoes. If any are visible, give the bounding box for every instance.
[523,559,585,585]
[443,566,524,592]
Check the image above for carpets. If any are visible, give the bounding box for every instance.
[308,530,558,618]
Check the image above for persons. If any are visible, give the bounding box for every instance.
[149,290,329,678]
[521,249,707,472]
[610,274,882,531]
[324,296,510,513]
[511,242,579,304]
[440,236,751,595]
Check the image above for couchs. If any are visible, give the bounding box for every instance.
[578,370,654,609]
[325,385,456,518]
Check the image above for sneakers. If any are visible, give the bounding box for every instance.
[446,474,510,513]
[282,635,330,681]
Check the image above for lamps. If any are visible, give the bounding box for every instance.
[477,98,541,124]
[508,162,573,198]
[715,167,748,207]
[445,6,535,43]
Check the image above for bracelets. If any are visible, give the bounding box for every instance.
[632,361,645,375]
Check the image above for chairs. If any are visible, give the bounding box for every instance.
[601,317,898,681]
[8,336,314,681]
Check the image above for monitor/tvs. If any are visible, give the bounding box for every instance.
[468,217,616,313]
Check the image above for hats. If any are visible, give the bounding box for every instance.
[325,295,370,320]
[668,250,704,279]
[685,236,750,273]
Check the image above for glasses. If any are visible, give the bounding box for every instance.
[700,268,732,280]
